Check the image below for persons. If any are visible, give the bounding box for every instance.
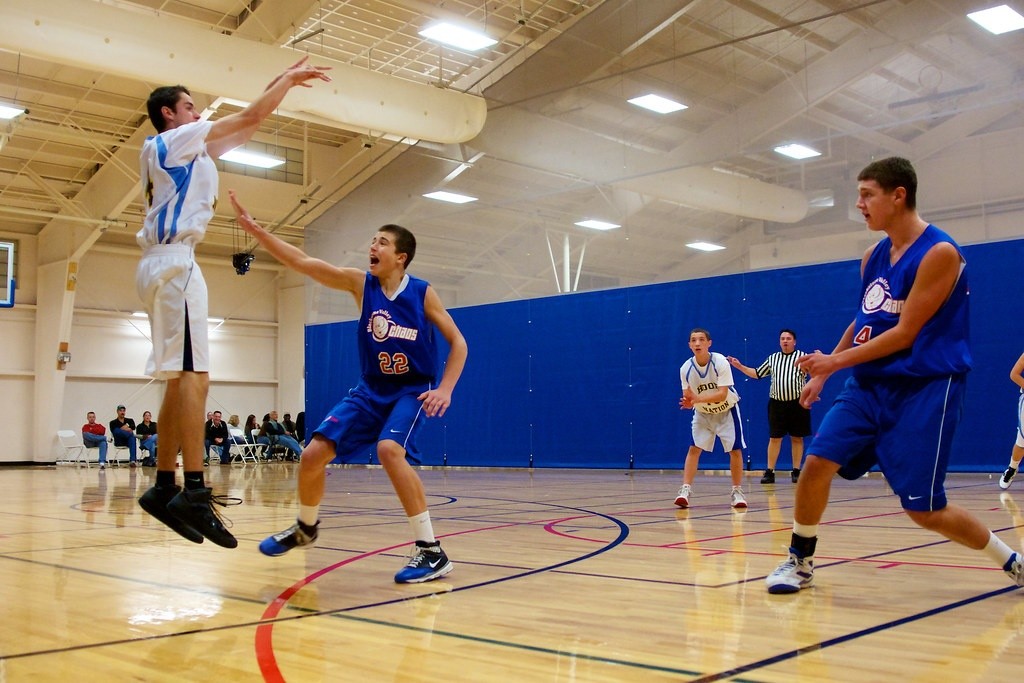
[82,412,113,469]
[726,328,822,485]
[110,405,143,467]
[204,410,230,465]
[999,353,1024,490]
[765,157,1024,594]
[261,411,305,461]
[226,414,246,462]
[136,411,159,467]
[673,328,748,508]
[136,54,333,549]
[228,189,468,583]
[245,414,272,463]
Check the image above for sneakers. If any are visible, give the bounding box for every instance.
[138,484,204,544]
[999,464,1019,490]
[394,540,453,583]
[732,507,747,515]
[673,486,694,507]
[166,487,242,549]
[791,468,801,483]
[258,518,321,556]
[675,508,689,524]
[766,547,814,594]
[761,469,776,483]
[731,487,747,507]
[1005,552,1024,587]
[1000,493,1019,516]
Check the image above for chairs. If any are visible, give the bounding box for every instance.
[229,429,260,464]
[138,439,150,464]
[57,429,90,471]
[111,432,129,467]
[74,438,111,468]
[251,429,272,463]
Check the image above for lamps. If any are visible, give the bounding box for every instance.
[418,0,499,52]
[0,54,30,121]
[218,108,287,169]
[620,0,689,114]
